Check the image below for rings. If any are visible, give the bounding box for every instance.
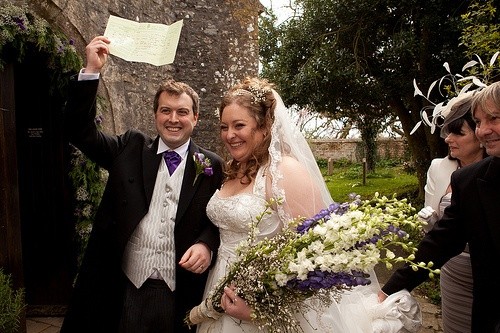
[200,265,204,270]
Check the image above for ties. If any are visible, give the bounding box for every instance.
[163,151,182,176]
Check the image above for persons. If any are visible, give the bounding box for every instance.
[194,81,423,333]
[59,36,226,333]
[372,50,500,333]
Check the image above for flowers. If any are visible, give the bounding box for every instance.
[184,191,441,333]
[192,151,214,188]
